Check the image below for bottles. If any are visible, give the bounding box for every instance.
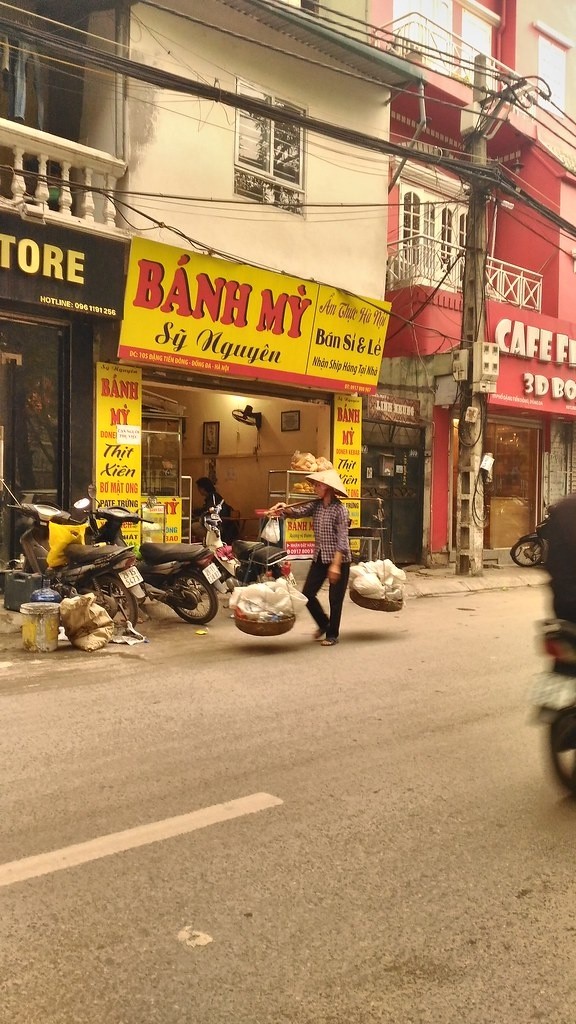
[30,579,62,603]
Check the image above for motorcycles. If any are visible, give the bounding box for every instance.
[192,494,297,594]
[532,619,576,802]
[509,498,557,566]
[83,482,227,624]
[0,479,145,627]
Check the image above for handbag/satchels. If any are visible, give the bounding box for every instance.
[45,521,86,568]
[261,514,280,544]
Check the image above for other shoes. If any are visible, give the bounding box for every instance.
[314,622,332,638]
[321,637,339,646]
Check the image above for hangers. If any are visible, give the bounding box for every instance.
[12,18,36,27]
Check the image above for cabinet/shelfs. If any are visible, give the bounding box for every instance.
[141,475,192,545]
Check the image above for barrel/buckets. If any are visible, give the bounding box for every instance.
[20,602,60,652]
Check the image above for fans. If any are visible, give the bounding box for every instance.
[232,405,262,448]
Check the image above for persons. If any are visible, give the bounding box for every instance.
[270,469,352,647]
[195,475,238,543]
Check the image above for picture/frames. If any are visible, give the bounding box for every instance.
[280,410,300,431]
[203,421,220,454]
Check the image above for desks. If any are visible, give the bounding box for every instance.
[220,516,262,543]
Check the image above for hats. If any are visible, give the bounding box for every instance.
[304,469,348,497]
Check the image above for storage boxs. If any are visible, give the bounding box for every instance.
[4,571,42,613]
